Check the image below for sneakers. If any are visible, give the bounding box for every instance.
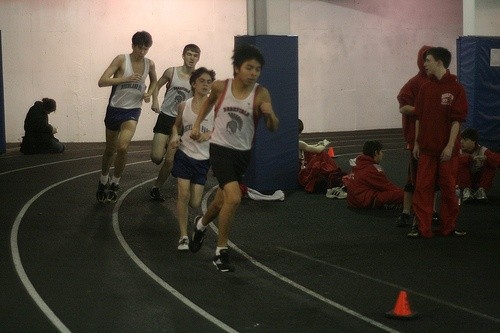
[96,180,109,203]
[212,248,234,273]
[326,187,348,199]
[106,182,120,203]
[189,216,207,254]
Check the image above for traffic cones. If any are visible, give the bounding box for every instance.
[383,290,419,320]
[328,147,334,157]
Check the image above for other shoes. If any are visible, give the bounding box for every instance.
[149,186,161,200]
[462,187,476,202]
[473,187,489,202]
[407,224,421,239]
[394,212,412,227]
[432,211,440,227]
[177,236,190,251]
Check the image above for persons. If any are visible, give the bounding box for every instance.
[393,44,469,236]
[20,97,64,155]
[346,140,405,209]
[459,126,500,202]
[297,119,348,199]
[171,68,216,251]
[96,30,158,204]
[189,47,279,273]
[150,44,201,198]
